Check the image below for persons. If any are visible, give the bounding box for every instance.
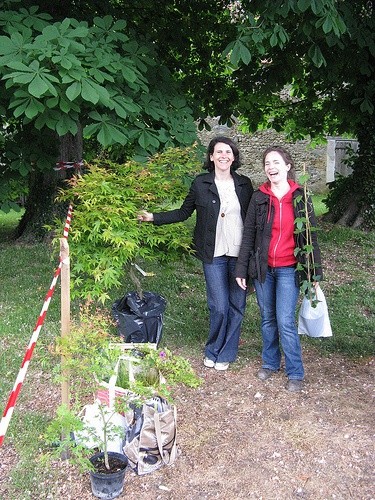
[233,146,323,391]
[137,137,255,370]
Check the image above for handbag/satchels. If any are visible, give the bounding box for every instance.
[92,355,162,419]
[121,396,178,475]
[73,402,127,454]
[297,283,333,338]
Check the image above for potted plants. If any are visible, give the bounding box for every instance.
[294,169,333,338]
[11,146,204,499]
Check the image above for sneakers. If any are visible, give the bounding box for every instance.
[288,379,301,392]
[214,361,229,370]
[257,368,273,379]
[203,357,214,368]
